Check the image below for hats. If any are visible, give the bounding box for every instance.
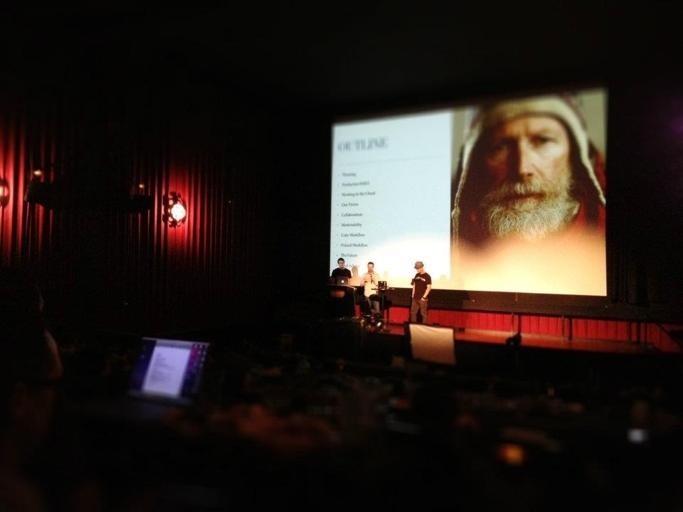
[415,262,424,269]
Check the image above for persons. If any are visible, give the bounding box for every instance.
[410,262,432,324]
[1,282,683,511]
[360,262,380,318]
[331,257,353,285]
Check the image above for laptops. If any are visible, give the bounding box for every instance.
[111,337,211,421]
